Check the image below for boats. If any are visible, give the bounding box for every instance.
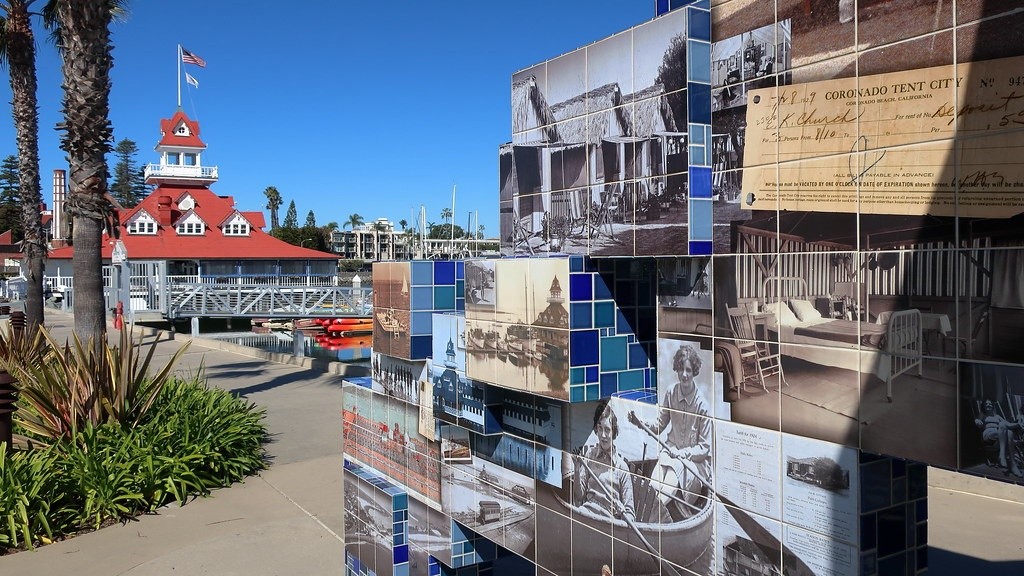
[377,309,404,335]
[534,456,713,576]
[467,322,547,362]
[259,317,373,336]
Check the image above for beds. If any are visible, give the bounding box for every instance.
[761,276,926,407]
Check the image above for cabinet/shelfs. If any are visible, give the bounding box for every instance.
[815,294,847,320]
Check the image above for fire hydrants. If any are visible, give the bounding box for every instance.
[116,299,125,329]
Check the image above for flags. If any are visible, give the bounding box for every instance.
[186,73,199,89]
[180,45,206,68]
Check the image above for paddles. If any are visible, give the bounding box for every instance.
[579,451,682,576]
[629,411,817,576]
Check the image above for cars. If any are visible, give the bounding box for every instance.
[512,484,530,501]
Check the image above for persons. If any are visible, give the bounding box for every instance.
[571,404,636,523]
[760,56,773,80]
[972,396,1024,479]
[1016,405,1024,433]
[627,346,715,509]
[386,306,399,323]
[601,565,612,576]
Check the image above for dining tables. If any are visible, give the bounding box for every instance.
[877,308,950,366]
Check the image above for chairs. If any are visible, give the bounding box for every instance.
[940,308,989,375]
[725,301,792,399]
